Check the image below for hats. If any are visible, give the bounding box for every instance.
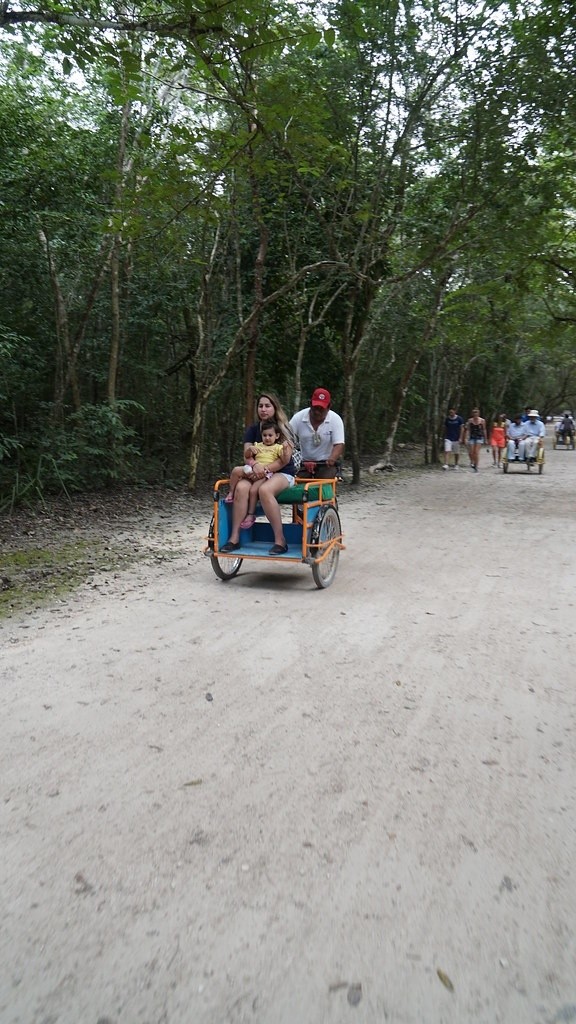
[311,388,330,409]
[527,410,539,417]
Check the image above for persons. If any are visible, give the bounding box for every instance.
[489,406,546,468]
[289,388,345,479]
[554,414,576,443]
[224,421,291,529]
[219,393,302,555]
[465,408,489,470]
[442,408,465,470]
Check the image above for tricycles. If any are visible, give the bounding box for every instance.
[202,460,346,589]
[552,428,576,451]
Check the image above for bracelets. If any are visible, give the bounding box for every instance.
[284,453,290,456]
[252,463,258,470]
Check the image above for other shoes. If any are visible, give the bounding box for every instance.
[525,456,536,463]
[221,541,240,553]
[520,460,525,464]
[493,464,497,468]
[453,465,459,470]
[508,459,513,463]
[471,464,478,471]
[443,465,450,471]
[498,464,503,469]
[269,544,288,554]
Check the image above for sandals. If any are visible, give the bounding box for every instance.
[240,513,256,529]
[225,496,233,503]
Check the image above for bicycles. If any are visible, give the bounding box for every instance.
[503,434,546,475]
